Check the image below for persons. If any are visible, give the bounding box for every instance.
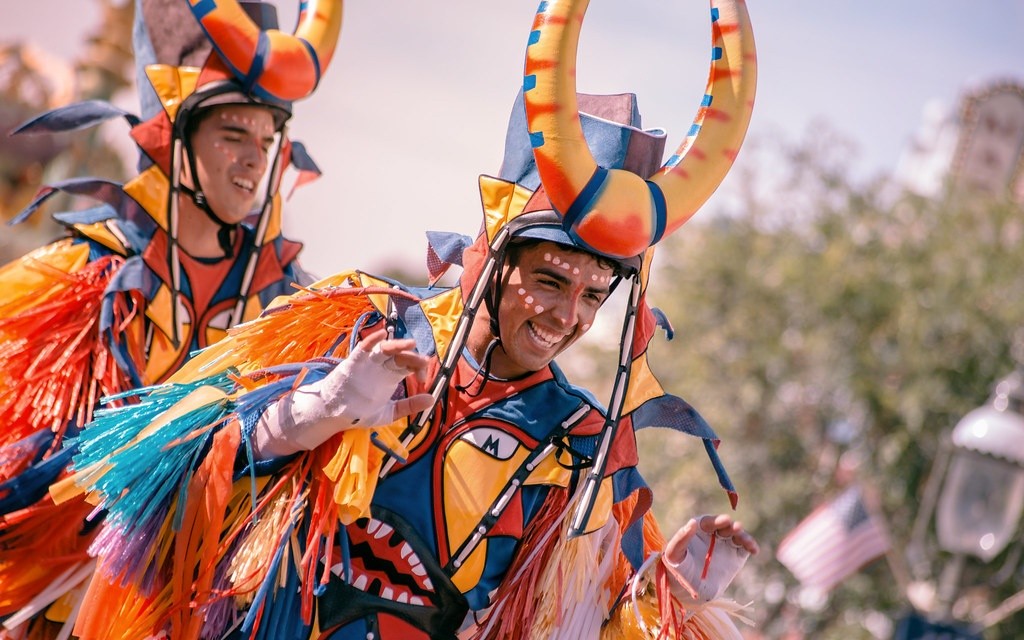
[72,89,760,640]
[0,0,345,640]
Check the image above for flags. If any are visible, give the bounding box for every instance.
[774,485,894,594]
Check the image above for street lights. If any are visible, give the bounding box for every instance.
[930,407,1024,622]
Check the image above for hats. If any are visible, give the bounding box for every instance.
[479,83,665,286]
[134,1,292,178]
